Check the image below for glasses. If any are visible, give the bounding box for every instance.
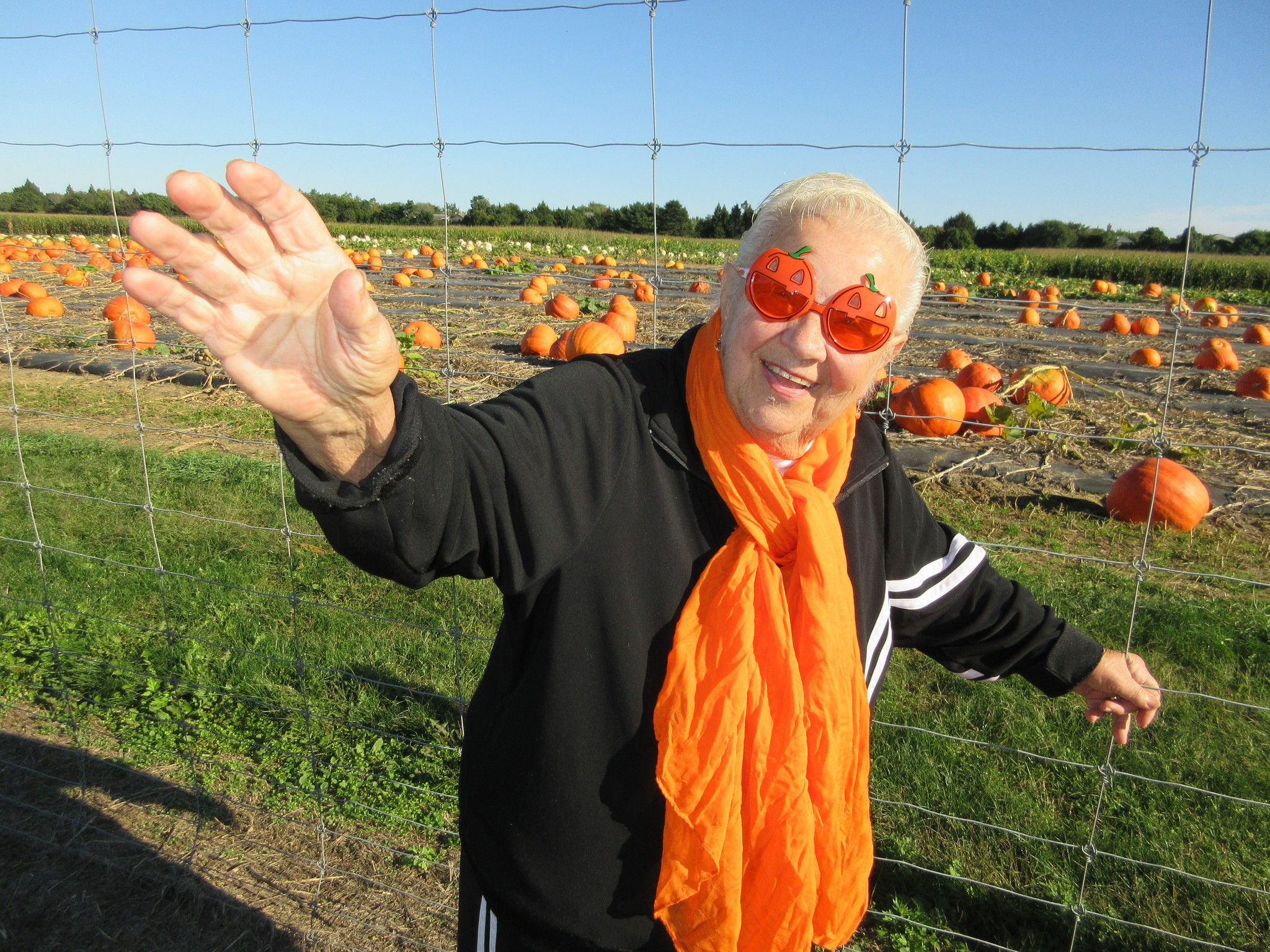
[727,246,909,353]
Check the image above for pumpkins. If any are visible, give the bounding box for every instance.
[565,321,624,362]
[871,272,1270,437]
[402,321,441,348]
[1106,457,1210,532]
[107,319,156,351]
[337,235,532,292]
[520,241,740,341]
[549,330,573,362]
[520,324,557,357]
[1,238,193,323]
[399,351,405,373]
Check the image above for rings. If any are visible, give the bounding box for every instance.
[1109,696,1119,700]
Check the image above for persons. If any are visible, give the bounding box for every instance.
[104,170,1165,952]
